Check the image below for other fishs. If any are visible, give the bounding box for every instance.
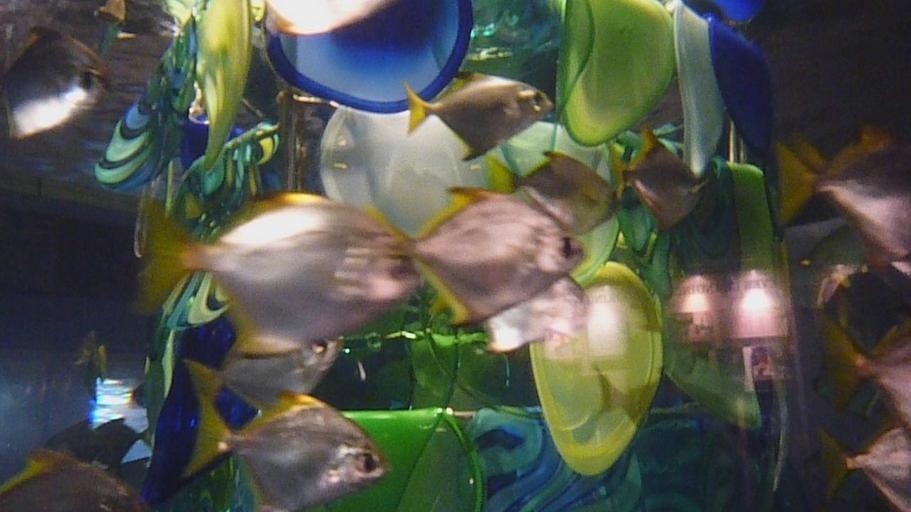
[0,0,911,510]
